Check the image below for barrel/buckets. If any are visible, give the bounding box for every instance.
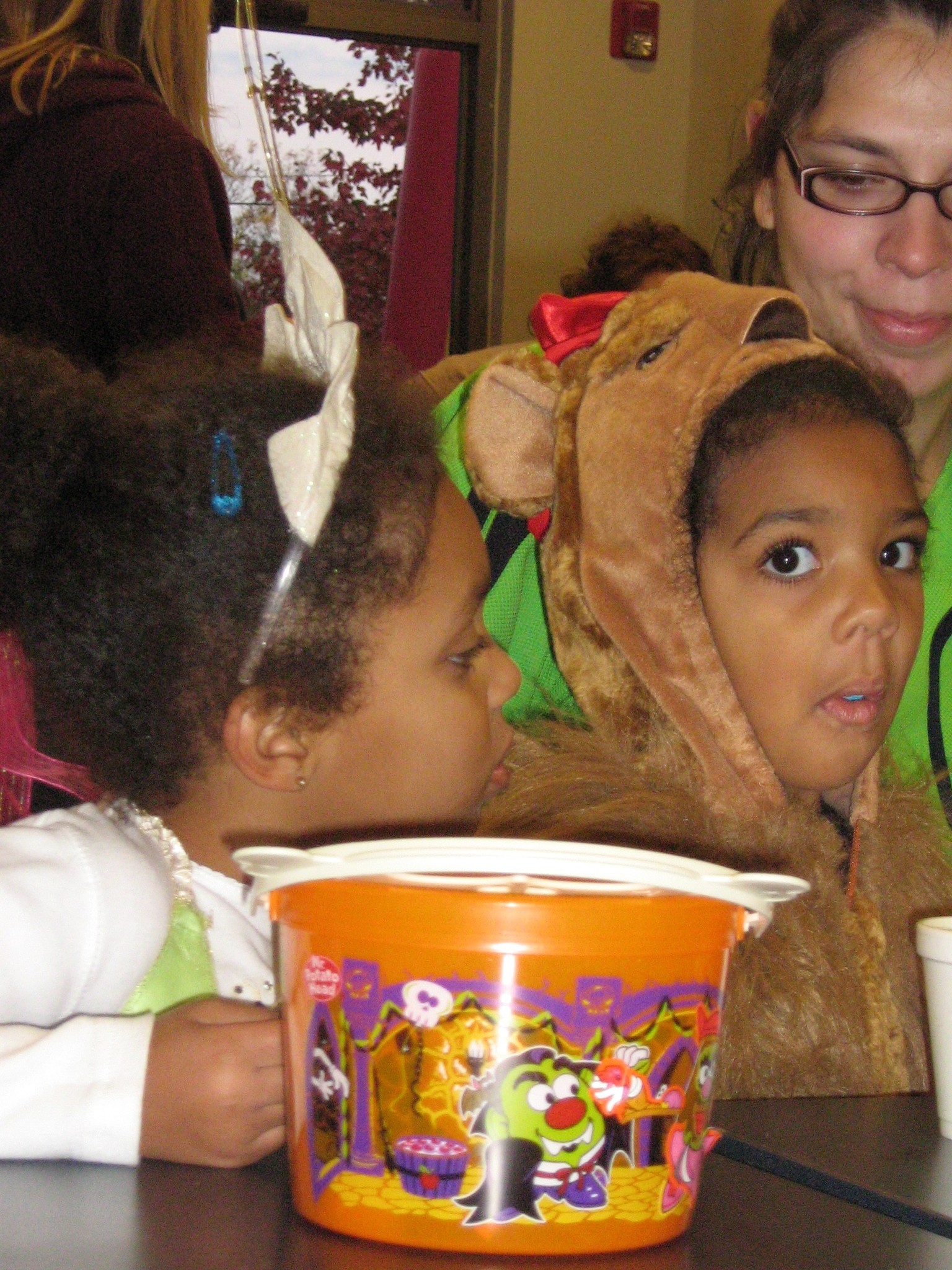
[222,832,817,1257]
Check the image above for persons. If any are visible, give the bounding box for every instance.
[0,0,278,373]
[0,332,525,1177]
[457,267,951,1104]
[719,3,951,927]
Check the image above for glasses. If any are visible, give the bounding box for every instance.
[774,128,952,219]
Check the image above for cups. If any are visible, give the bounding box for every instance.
[913,913,952,1141]
